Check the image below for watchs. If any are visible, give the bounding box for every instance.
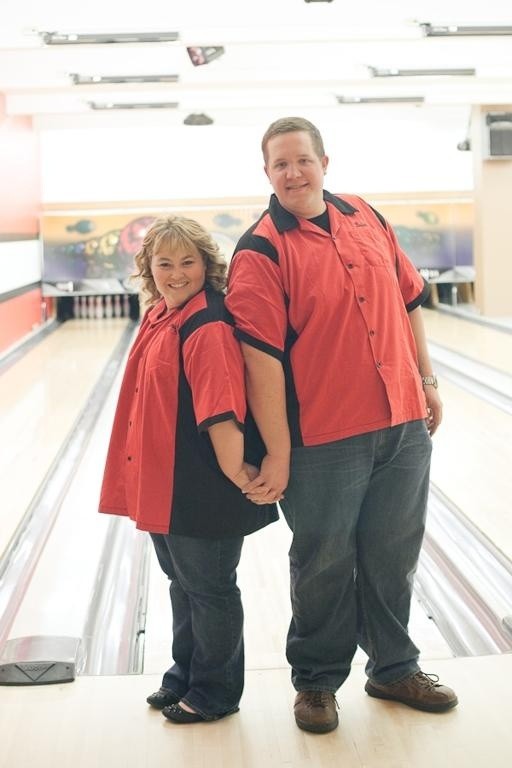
[421,375,439,388]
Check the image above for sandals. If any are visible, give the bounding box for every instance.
[146,688,205,723]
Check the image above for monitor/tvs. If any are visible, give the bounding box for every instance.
[487,125,512,161]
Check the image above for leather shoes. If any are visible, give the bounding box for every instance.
[293,685,338,732]
[365,670,458,713]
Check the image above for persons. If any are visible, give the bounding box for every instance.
[97,215,284,723]
[223,116,459,735]
